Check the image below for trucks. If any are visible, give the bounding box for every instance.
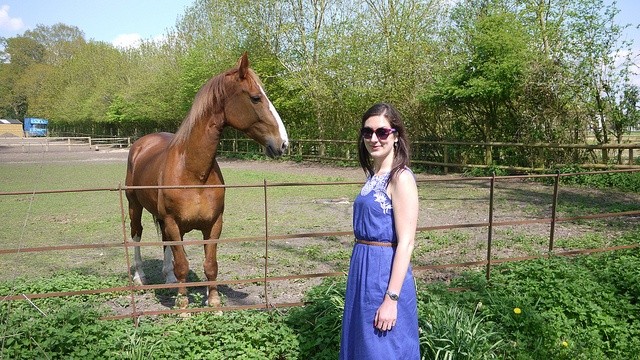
[24,118,48,137]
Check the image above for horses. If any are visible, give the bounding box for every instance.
[123,48,291,321]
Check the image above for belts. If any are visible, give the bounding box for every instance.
[353,238,395,247]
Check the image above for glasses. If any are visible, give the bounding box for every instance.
[359,127,396,139]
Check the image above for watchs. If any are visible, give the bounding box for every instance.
[386,290,399,302]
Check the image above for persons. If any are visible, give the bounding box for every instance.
[341,103,421,360]
